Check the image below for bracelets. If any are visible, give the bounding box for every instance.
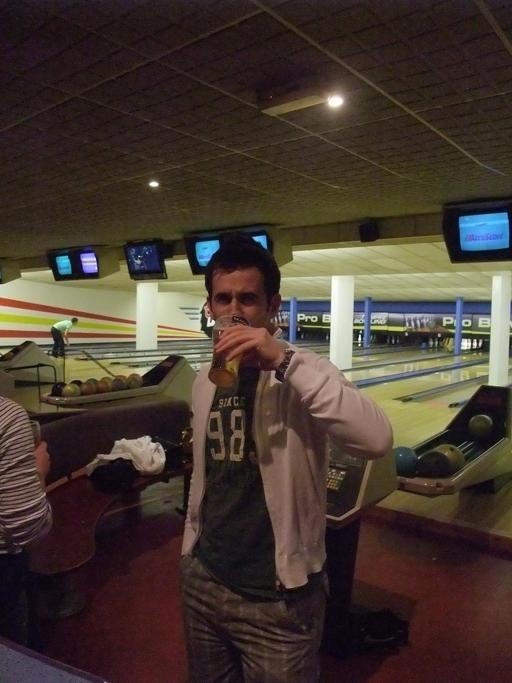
[274,348,295,383]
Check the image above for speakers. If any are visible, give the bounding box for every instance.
[359,222,379,242]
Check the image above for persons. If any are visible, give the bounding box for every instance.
[51,317,79,359]
[180,234,394,681]
[0,396,56,636]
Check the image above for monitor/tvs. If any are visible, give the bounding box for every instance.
[45,248,77,282]
[123,238,168,281]
[442,198,512,264]
[183,229,227,275]
[74,244,120,280]
[230,224,294,270]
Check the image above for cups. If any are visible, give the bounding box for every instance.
[30,420,41,448]
[207,314,250,388]
